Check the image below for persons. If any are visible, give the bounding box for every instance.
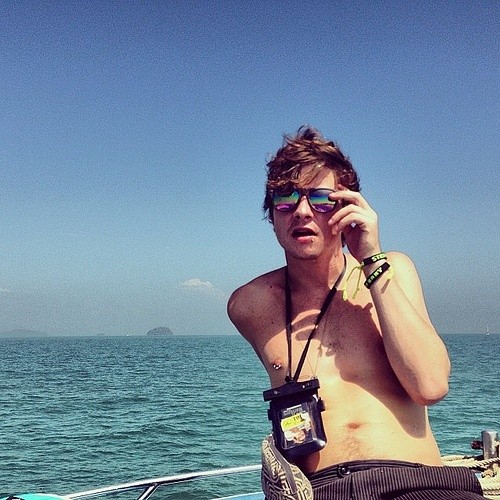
[228,123,492,500]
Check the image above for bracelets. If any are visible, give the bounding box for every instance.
[342,250,390,301]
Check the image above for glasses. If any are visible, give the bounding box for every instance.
[271,186,338,213]
